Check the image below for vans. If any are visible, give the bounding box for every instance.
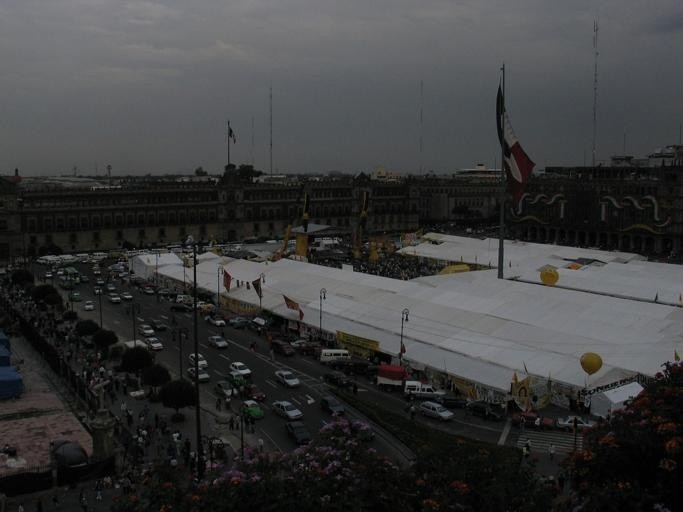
[320,349,351,362]
[36,244,242,264]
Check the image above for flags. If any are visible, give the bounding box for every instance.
[495,77,536,201]
[229,125,236,144]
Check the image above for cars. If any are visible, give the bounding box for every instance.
[186,353,267,420]
[42,264,217,313]
[271,339,329,360]
[512,412,597,432]
[404,380,505,422]
[271,396,345,446]
[138,314,251,351]
[274,370,300,387]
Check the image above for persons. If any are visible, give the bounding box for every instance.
[569,240,678,263]
[1,274,273,512]
[335,377,611,492]
[306,243,449,280]
